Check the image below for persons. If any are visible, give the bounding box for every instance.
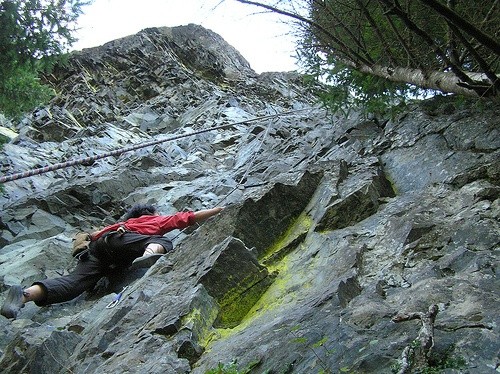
[0,202,227,320]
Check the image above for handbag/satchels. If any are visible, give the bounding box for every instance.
[71,232,95,262]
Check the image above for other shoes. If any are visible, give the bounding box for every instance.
[132,253,166,269]
[0,285,26,321]
